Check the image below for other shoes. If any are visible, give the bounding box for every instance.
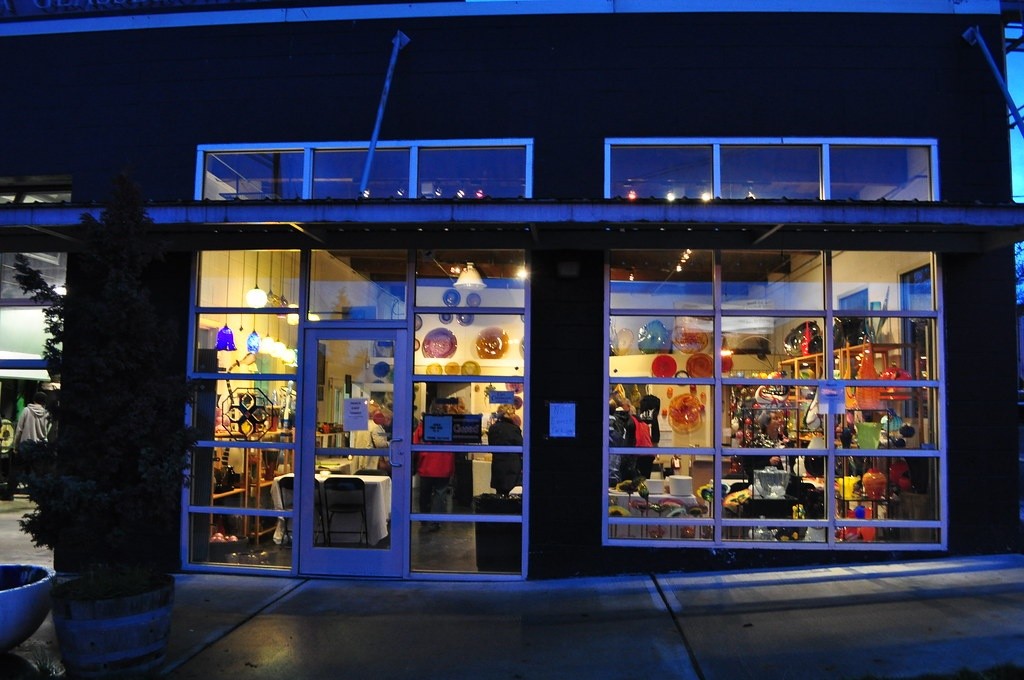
[421,523,439,533]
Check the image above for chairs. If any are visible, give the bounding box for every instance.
[278,468,386,549]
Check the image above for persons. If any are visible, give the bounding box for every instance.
[741,413,813,499]
[14,392,52,495]
[412,397,473,532]
[353,401,393,471]
[609,395,660,486]
[488,404,523,495]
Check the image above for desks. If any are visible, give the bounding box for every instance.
[270,473,391,545]
[609,476,888,542]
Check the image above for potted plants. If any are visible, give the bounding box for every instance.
[0,174,207,680]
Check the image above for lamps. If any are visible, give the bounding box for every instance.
[216,249,321,366]
[453,262,488,292]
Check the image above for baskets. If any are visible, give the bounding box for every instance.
[841,335,880,409]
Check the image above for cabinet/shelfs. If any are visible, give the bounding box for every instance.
[210,428,351,559]
[779,343,916,543]
